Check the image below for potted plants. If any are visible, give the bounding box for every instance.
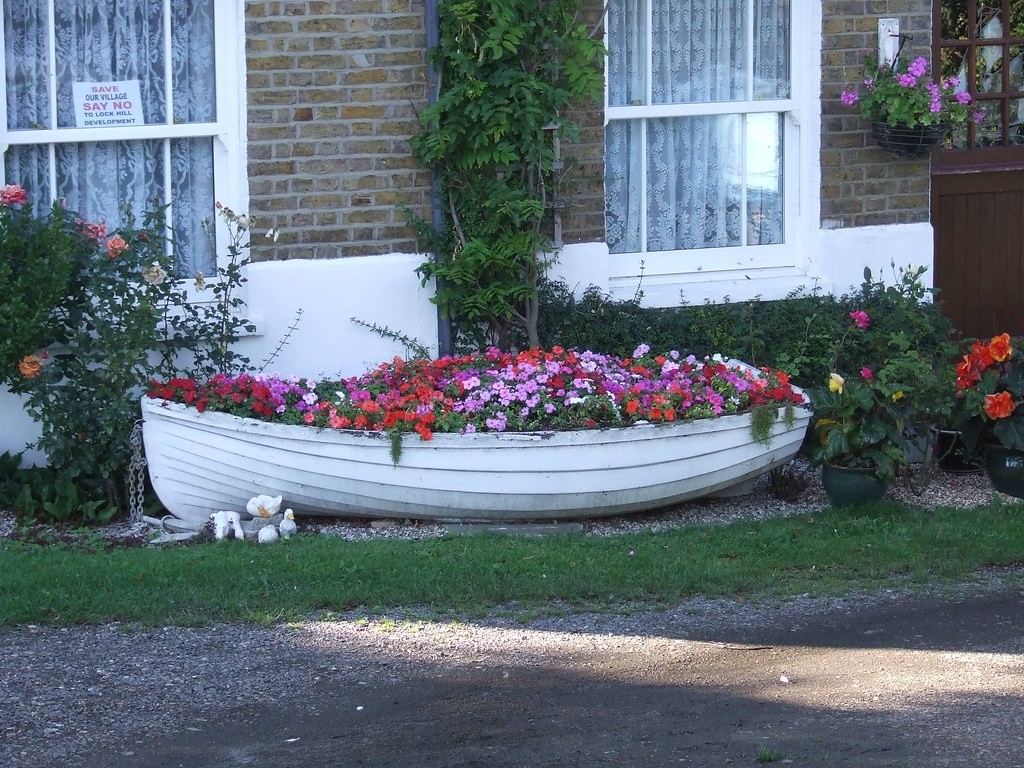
[881,353,986,473]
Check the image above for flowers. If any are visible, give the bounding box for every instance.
[955,333,1024,455]
[809,309,918,481]
[841,51,986,137]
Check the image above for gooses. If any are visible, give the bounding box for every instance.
[210,495,297,544]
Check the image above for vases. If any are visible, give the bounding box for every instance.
[820,463,888,509]
[872,121,948,161]
[984,440,1024,500]
